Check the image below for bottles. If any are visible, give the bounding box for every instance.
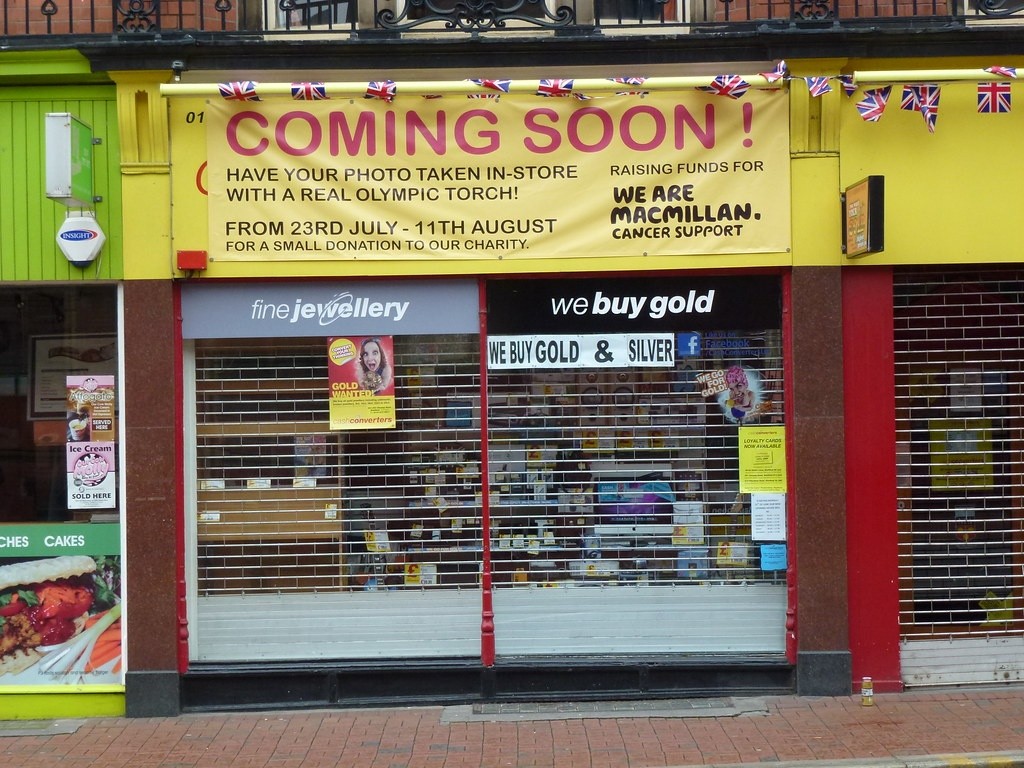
[862,677,873,705]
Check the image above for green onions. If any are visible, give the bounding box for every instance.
[34,600,121,684]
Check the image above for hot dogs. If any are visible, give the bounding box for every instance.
[0,555,97,676]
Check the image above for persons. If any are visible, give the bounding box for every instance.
[726,367,756,426]
[361,339,391,393]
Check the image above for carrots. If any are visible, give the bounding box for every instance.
[85,609,121,674]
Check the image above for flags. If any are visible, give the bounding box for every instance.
[465,66,1020,135]
[363,79,397,104]
[291,80,331,100]
[220,80,264,102]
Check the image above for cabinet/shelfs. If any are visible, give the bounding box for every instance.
[195,337,348,596]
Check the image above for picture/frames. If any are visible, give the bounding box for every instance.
[27,333,117,421]
